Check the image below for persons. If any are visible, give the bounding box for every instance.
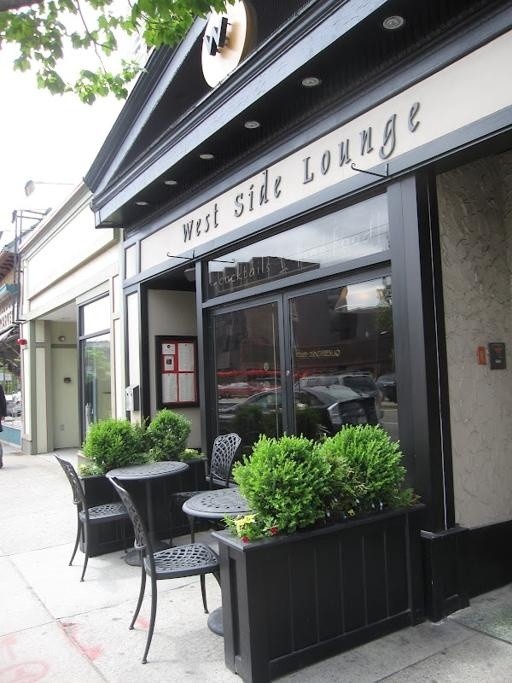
[0,382,7,469]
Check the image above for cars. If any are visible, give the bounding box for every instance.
[218,373,397,434]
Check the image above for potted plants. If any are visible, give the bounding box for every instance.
[214,425,427,682]
[77,407,210,557]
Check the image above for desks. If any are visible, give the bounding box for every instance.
[105,461,189,567]
[181,486,257,639]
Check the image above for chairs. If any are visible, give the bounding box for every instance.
[55,455,143,581]
[169,432,241,550]
[108,475,223,665]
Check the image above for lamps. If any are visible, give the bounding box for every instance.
[12,179,80,224]
